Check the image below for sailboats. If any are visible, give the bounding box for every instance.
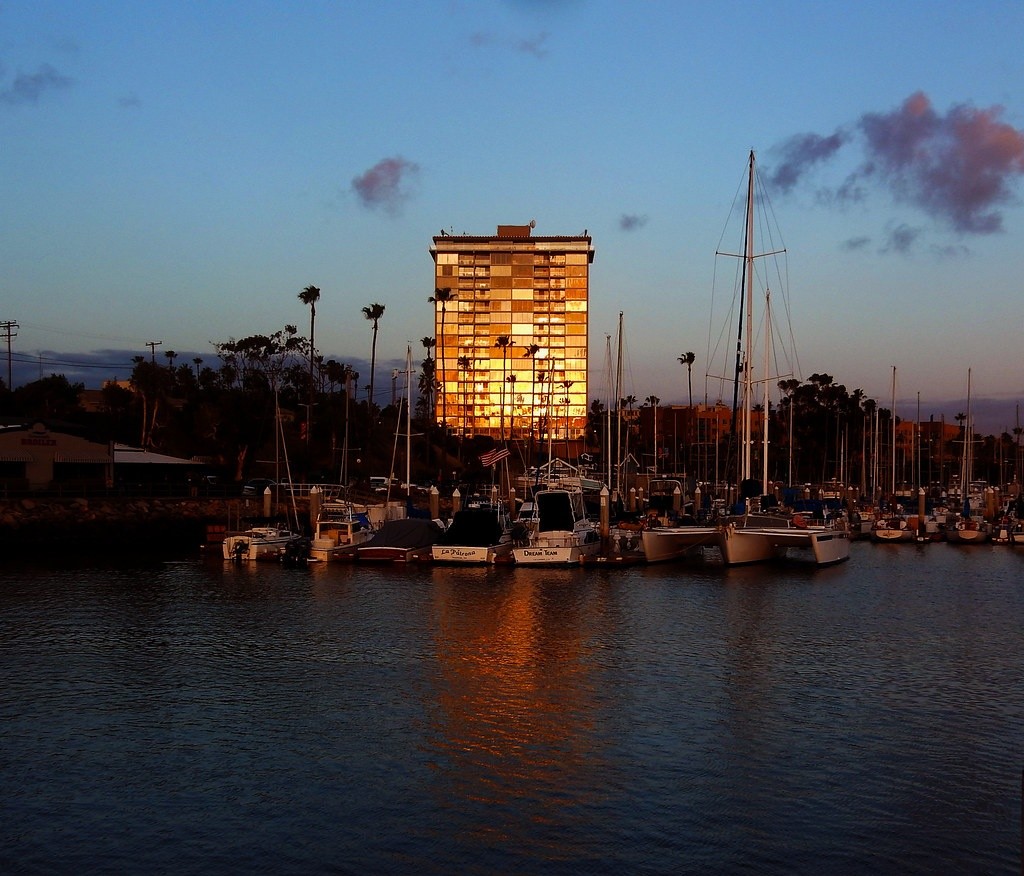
[222,145,1024,572]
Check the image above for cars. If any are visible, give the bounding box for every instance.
[206,476,389,504]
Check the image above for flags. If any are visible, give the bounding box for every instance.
[479,441,511,467]
